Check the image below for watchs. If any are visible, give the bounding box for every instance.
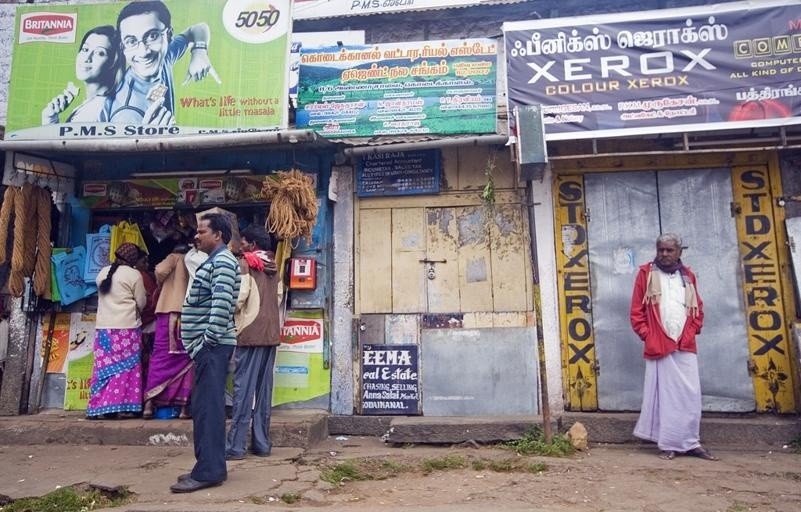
[190,41,209,52]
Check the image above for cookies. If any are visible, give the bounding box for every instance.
[67,82,80,96]
[108,182,132,205]
[226,178,242,200]
[147,84,168,101]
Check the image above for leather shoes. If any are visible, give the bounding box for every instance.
[171,473,222,492]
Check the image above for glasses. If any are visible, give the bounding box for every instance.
[120,26,168,50]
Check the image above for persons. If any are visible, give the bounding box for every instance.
[41,25,126,126]
[110,0,222,126]
[631,233,719,461]
[137,250,154,291]
[224,223,282,459]
[85,243,147,422]
[170,213,242,493]
[143,243,194,420]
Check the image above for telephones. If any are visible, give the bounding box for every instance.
[283,257,316,289]
